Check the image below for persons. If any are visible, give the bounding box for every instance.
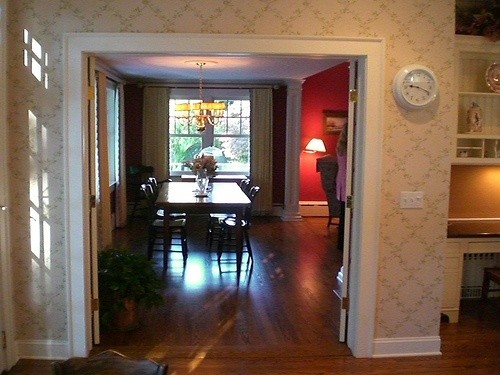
[336,122,348,250]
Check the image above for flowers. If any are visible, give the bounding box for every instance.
[455,0,500,41]
[184,154,218,180]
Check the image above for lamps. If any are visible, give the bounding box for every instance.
[174,60,226,131]
[304,138,326,153]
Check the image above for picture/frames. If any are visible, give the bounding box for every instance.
[322,112,348,133]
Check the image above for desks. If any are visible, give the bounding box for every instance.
[157,181,252,275]
[180,174,248,184]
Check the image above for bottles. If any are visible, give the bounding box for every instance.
[467,103,483,134]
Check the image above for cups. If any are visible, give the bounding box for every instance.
[491,139,500,157]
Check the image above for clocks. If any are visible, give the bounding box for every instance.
[392,63,440,109]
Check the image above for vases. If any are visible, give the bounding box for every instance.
[195,168,210,194]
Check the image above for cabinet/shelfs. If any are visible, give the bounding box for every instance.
[442,233,500,324]
[452,36,500,167]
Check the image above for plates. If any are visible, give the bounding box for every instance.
[485,61,500,92]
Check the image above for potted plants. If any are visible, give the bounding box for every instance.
[97,243,165,334]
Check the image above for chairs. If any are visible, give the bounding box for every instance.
[316,154,340,227]
[206,178,250,251]
[480,267,500,321]
[127,164,153,222]
[139,177,188,267]
[214,185,261,263]
